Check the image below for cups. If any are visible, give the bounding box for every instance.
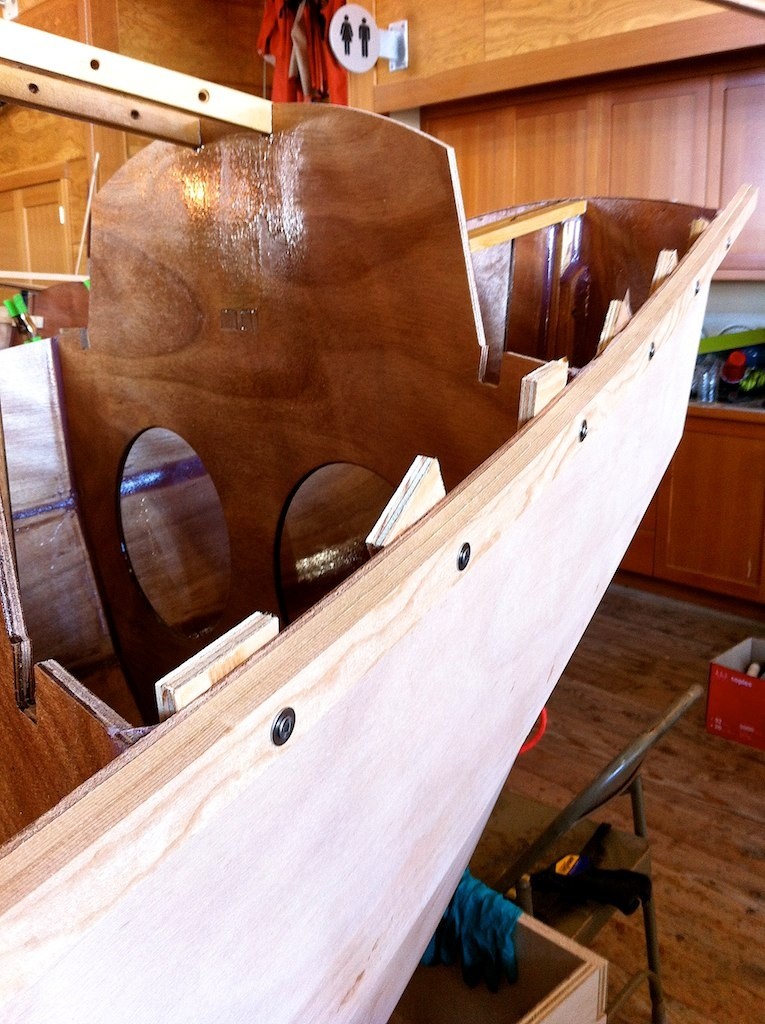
[696,355,719,403]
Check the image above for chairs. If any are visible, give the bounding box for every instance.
[467,681,706,1024]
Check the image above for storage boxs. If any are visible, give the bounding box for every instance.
[706,635,765,749]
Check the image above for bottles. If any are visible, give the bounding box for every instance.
[718,351,746,404]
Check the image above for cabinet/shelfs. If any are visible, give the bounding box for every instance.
[617,401,765,603]
